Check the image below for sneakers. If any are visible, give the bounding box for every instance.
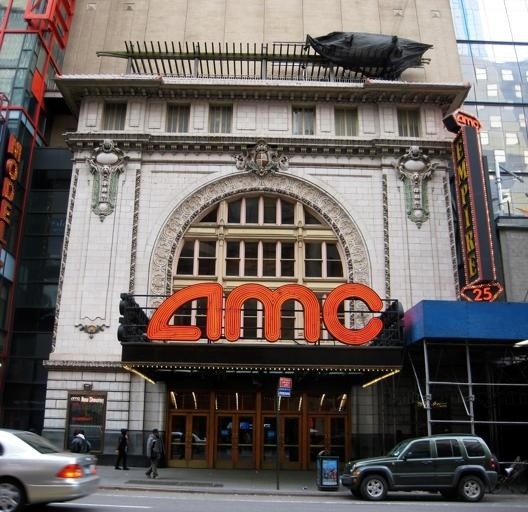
[145,472,158,479]
[115,466,130,470]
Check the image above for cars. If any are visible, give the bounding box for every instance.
[0,428,100,512]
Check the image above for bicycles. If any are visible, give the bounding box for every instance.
[489,456,528,494]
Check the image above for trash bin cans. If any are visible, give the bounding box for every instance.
[317,449,340,491]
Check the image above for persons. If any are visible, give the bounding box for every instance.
[68,428,92,454]
[114,428,131,470]
[144,428,166,480]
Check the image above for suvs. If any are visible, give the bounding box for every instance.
[340,433,499,502]
